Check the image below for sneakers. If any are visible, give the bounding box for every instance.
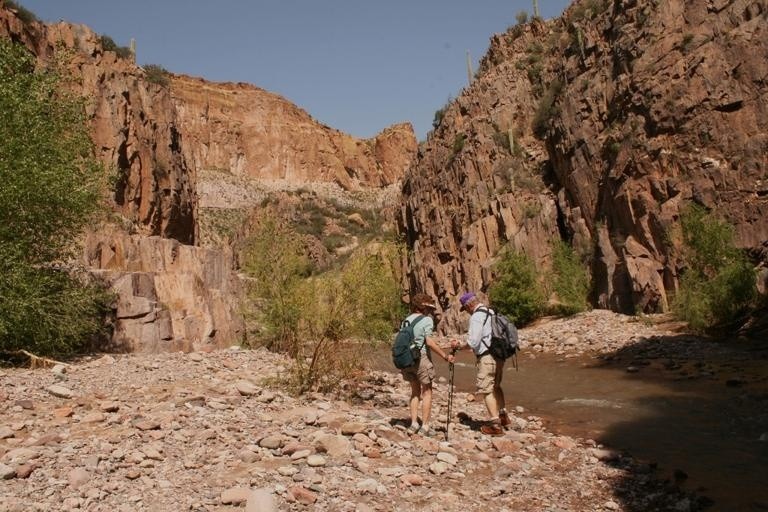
[480,413,510,436]
[405,424,437,437]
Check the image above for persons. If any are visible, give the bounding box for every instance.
[450,291,513,438]
[399,292,455,438]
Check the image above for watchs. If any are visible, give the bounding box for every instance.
[456,341,461,350]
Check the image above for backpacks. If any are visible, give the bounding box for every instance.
[391,314,428,369]
[475,308,518,359]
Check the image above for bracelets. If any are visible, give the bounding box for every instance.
[444,353,448,362]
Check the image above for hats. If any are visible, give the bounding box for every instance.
[459,293,477,312]
[411,293,436,310]
